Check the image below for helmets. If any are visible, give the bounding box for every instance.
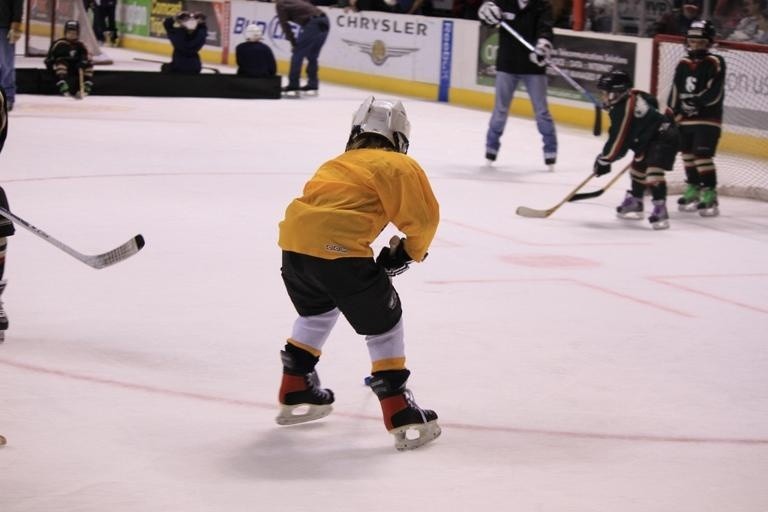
[245,24,261,40]
[64,19,81,40]
[684,20,717,59]
[350,98,411,155]
[597,71,630,109]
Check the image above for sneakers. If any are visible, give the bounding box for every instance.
[487,146,495,159]
[544,152,556,163]
[282,86,298,91]
[302,85,317,90]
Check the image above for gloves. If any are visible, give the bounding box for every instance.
[595,154,610,175]
[479,2,502,27]
[531,39,554,66]
[375,239,411,276]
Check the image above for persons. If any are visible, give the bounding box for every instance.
[0,0,23,111]
[234,24,277,76]
[0,88,15,333]
[663,20,726,210]
[43,19,93,100]
[476,0,558,165]
[159,11,207,76]
[275,0,330,91]
[559,0,768,44]
[84,0,118,47]
[593,68,681,223]
[330,0,435,20]
[275,95,441,431]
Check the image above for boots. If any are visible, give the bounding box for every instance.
[649,199,668,222]
[677,180,718,209]
[278,350,334,405]
[365,370,438,433]
[618,196,644,214]
[0,280,9,329]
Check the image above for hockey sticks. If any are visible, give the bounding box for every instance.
[570,115,682,199]
[134,58,219,74]
[516,174,598,219]
[499,19,605,111]
[1,208,145,269]
